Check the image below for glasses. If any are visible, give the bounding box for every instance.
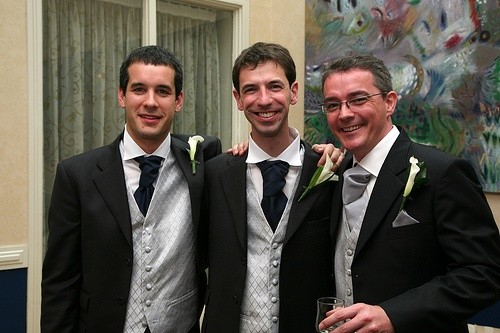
[319,91,388,114]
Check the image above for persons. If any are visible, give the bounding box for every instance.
[227,55,500,333]
[40,46,345,333]
[204,42,337,333]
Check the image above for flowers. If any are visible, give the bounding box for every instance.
[297,152,339,202]
[185,135,204,175]
[398,155,427,212]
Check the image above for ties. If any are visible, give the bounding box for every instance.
[342,165,372,231]
[134,155,165,217]
[256,160,290,233]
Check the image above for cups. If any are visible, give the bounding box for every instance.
[316,297,345,333]
